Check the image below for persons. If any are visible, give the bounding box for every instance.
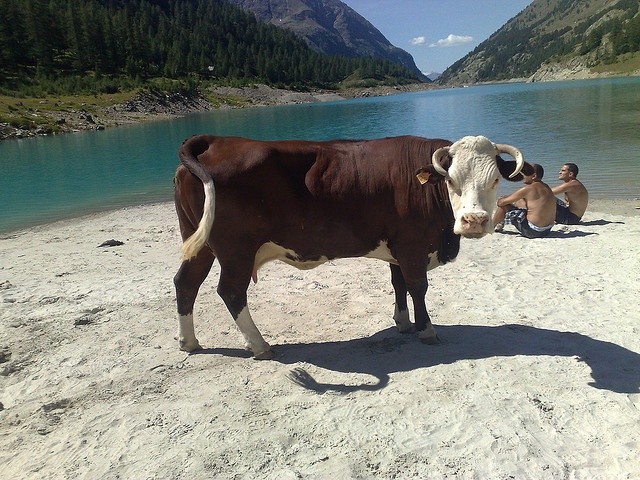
[550,163,589,225]
[491,163,557,239]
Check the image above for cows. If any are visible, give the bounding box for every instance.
[173,134,524,360]
